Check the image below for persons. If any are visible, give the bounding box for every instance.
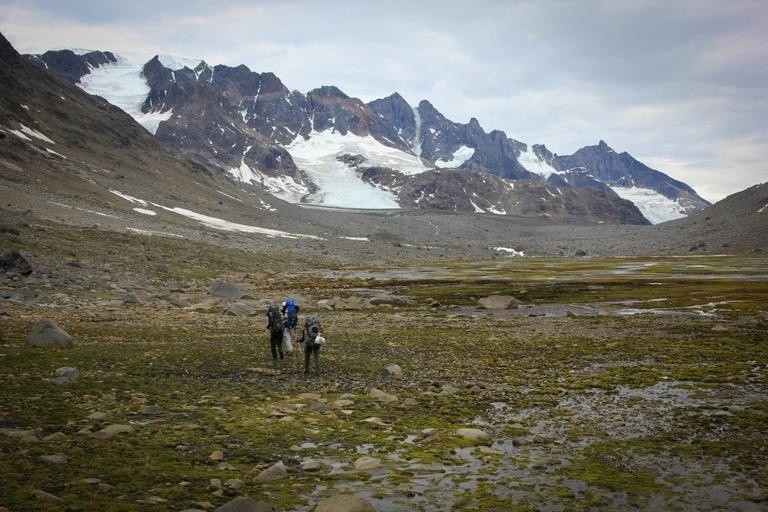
[265,300,285,360]
[296,314,327,375]
[281,299,302,355]
